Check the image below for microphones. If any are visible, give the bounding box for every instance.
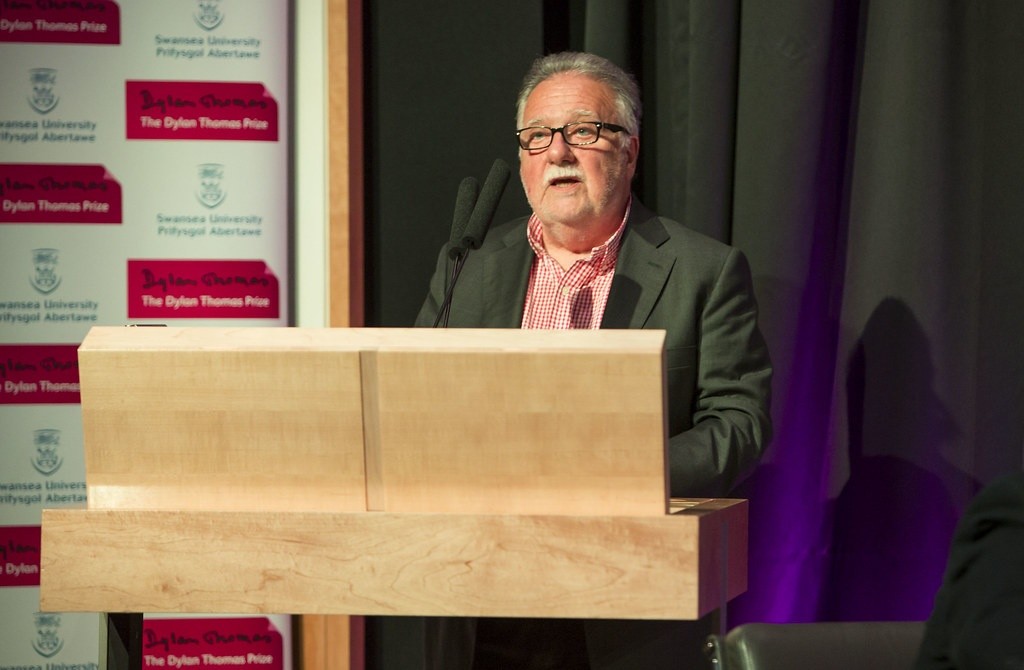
[432,159,511,329]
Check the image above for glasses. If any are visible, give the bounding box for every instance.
[517,121,631,151]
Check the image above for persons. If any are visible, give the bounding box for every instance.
[362,48,773,502]
